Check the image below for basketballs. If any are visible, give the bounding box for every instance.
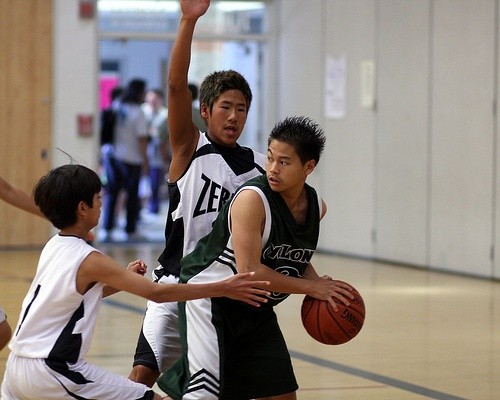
[300,280,366,345]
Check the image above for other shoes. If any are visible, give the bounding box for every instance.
[102,235,148,243]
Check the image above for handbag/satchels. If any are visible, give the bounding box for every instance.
[101,109,115,145]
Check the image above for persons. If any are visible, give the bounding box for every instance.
[157,114,354,400]
[0,176,52,351]
[0,165,271,400]
[99,77,210,243]
[126,0,267,389]
